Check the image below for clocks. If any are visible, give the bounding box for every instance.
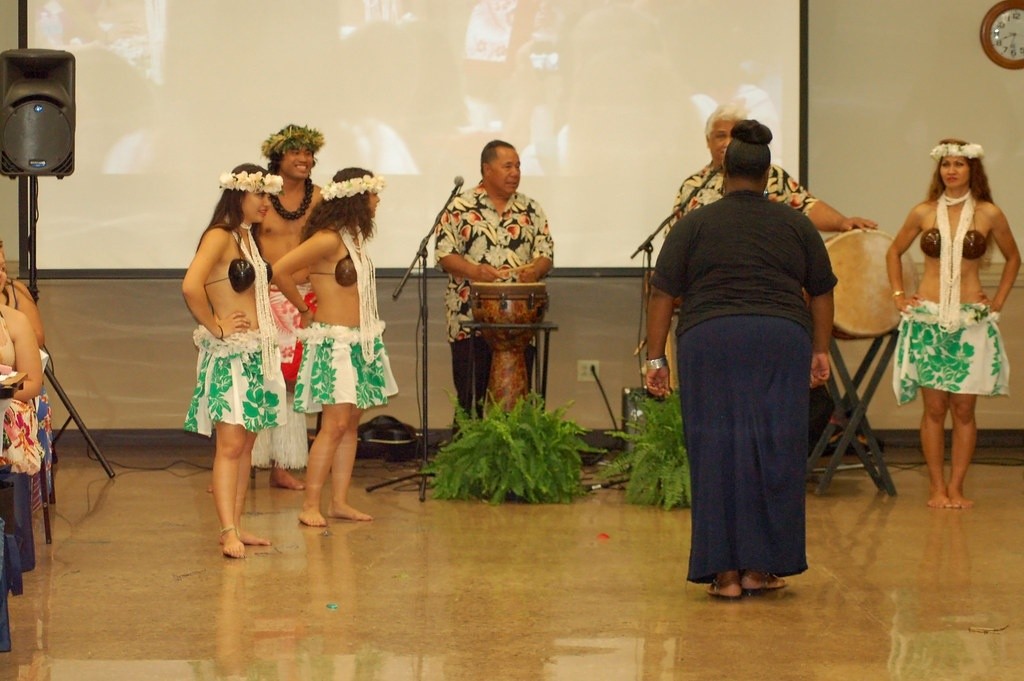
[980,0,1024,70]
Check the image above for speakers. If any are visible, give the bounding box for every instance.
[0,47,75,181]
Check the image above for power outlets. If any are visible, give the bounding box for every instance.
[576,360,601,383]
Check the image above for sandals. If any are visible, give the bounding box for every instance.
[743,573,787,598]
[707,578,743,601]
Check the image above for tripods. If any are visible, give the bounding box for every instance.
[27,176,116,504]
[365,187,461,502]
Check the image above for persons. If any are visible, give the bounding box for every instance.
[435,140,553,445]
[273,168,390,527]
[182,163,283,558]
[886,139,1021,509]
[0,241,45,651]
[645,105,878,599]
[207,124,331,493]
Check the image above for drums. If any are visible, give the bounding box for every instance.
[801,228,919,341]
[469,280,548,416]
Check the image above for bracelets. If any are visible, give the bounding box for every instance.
[216,323,224,340]
[297,304,309,313]
[892,290,905,297]
[646,355,666,370]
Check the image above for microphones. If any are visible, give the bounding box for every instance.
[455,177,465,186]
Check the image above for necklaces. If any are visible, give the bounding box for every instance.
[269,177,314,220]
